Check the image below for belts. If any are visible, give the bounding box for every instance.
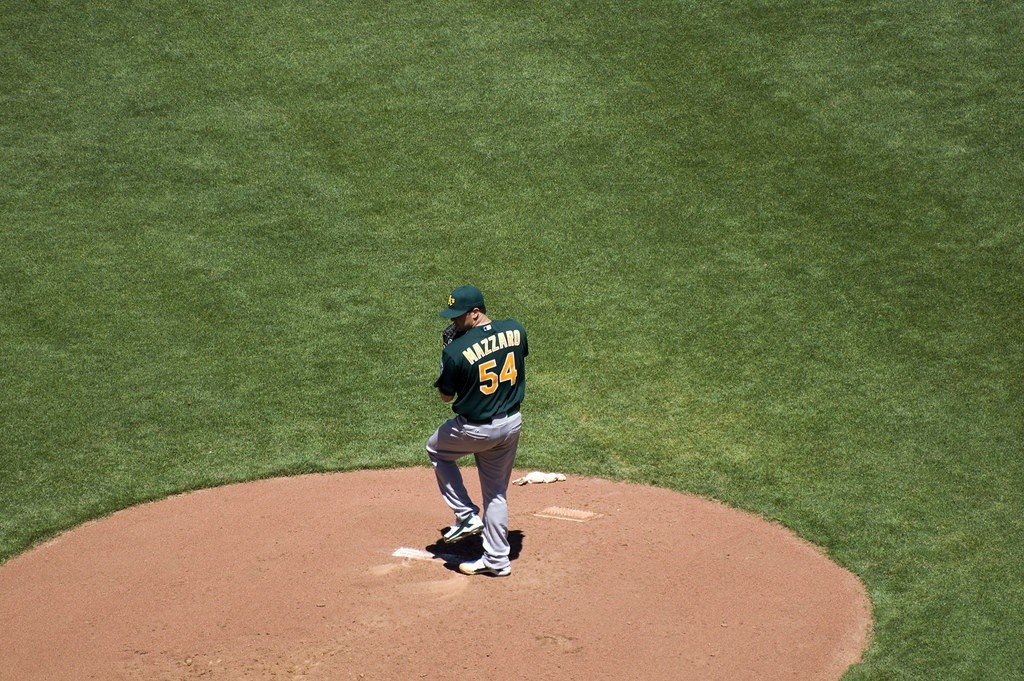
[467,403,520,425]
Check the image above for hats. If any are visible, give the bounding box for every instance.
[439,286,484,318]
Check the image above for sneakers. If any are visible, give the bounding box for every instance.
[443,514,484,544]
[459,558,511,575]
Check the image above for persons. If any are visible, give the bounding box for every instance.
[425,287,527,576]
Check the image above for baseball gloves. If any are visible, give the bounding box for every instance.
[442,323,460,348]
